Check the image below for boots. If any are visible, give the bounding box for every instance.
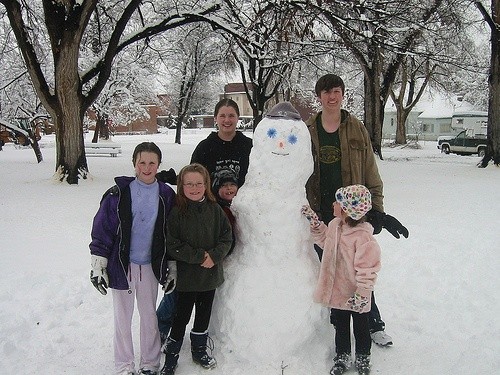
[161,337,182,375]
[190,329,217,369]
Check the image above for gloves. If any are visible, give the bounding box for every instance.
[366,209,385,235]
[346,294,369,312]
[90,255,110,295]
[382,214,409,239]
[300,205,321,228]
[162,260,177,294]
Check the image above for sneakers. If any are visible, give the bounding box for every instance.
[370,330,392,346]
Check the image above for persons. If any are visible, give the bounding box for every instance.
[90,141,177,375]
[190,98,253,205]
[300,183,381,375]
[211,169,238,209]
[162,163,233,375]
[306,74,409,348]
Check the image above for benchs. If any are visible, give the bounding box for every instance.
[85,143,122,157]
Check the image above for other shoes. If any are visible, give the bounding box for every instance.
[354,352,370,375]
[138,367,157,375]
[330,351,353,375]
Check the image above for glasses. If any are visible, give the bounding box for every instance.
[182,181,206,189]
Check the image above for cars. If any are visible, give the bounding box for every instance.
[436,129,488,157]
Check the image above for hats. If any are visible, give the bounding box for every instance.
[265,102,301,120]
[335,185,372,221]
[209,167,240,194]
